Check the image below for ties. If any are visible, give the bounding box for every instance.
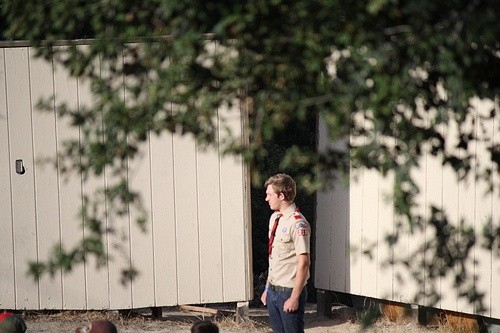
[268,214,283,255]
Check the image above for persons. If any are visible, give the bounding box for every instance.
[260,173,311,333]
[0,313,219,333]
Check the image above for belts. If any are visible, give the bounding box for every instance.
[269,282,289,292]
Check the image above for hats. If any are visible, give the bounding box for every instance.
[75,319,118,333]
[0,312,27,333]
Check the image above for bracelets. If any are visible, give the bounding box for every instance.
[265,287,269,291]
